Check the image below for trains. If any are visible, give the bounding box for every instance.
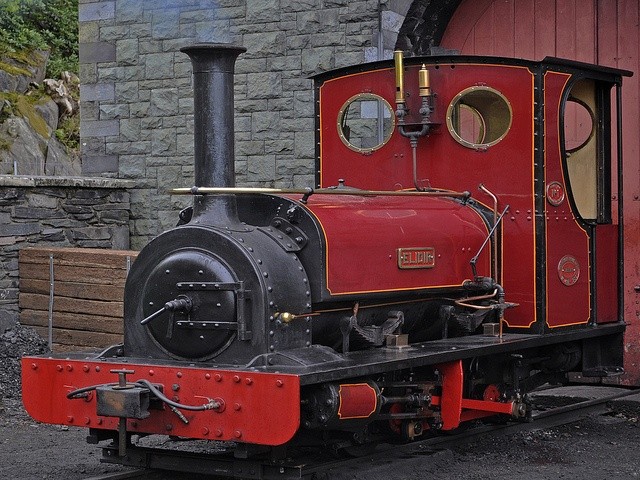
[20,40,639,479]
